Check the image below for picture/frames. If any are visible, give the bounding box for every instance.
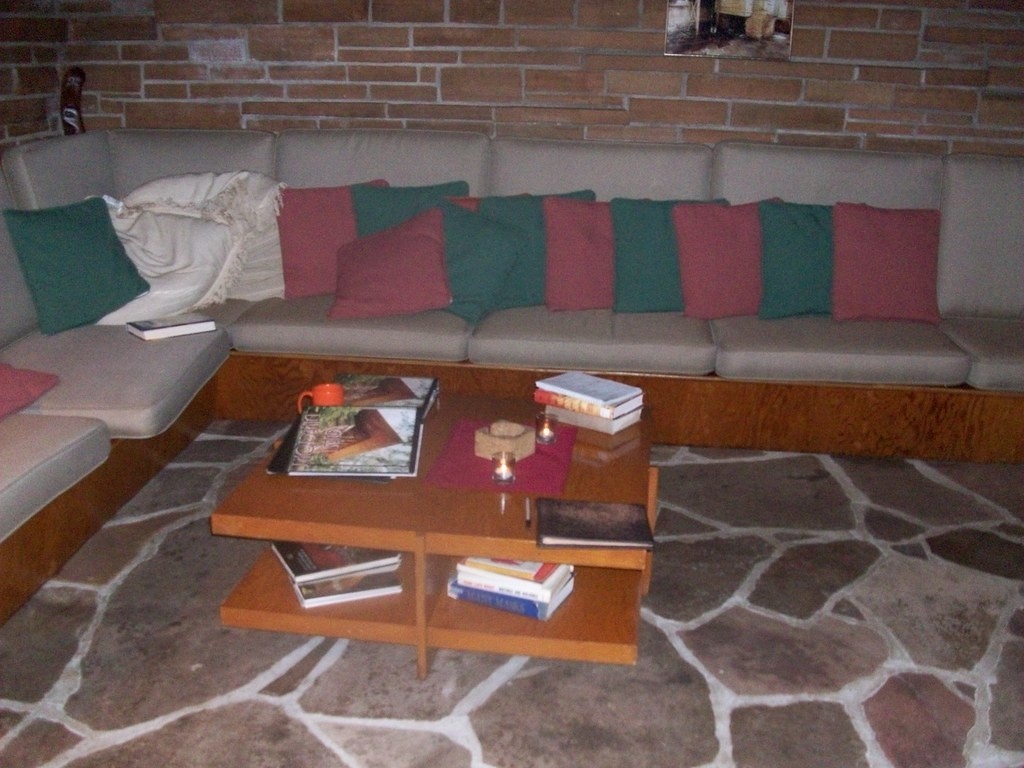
[663,0,795,63]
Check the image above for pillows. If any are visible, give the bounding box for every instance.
[672,196,783,321]
[477,187,595,309]
[610,196,730,312]
[277,179,390,299]
[542,193,613,309]
[757,200,833,321]
[416,189,532,326]
[327,208,453,322]
[445,192,529,212]
[833,200,942,327]
[351,181,469,239]
[1,361,118,424]
[2,196,151,337]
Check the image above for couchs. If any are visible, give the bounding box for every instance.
[0,127,1024,630]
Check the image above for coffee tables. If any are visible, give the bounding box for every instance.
[212,391,659,680]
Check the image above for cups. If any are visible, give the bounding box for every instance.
[535,412,559,445]
[493,451,515,484]
[298,384,344,417]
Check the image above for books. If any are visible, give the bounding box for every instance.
[448,556,575,622]
[266,373,440,484]
[534,371,647,435]
[271,542,403,609]
[126,312,217,341]
[536,498,654,550]
